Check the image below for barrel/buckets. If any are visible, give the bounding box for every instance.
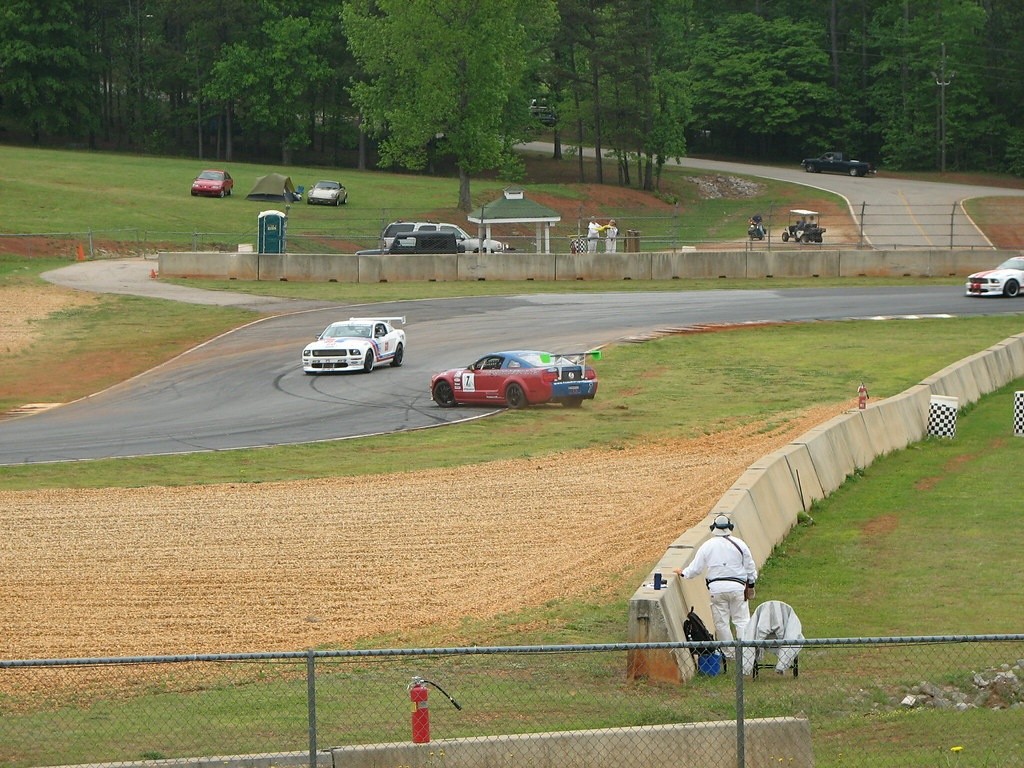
[698,650,721,677]
[238,244,253,251]
[681,246,696,252]
[298,186,304,194]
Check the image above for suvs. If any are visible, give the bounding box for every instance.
[355,220,502,255]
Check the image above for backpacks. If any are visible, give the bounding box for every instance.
[683,607,717,655]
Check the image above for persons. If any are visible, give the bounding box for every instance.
[794,216,807,234]
[673,514,758,658]
[825,154,833,160]
[604,219,618,252]
[588,216,608,253]
[807,216,816,224]
[753,212,765,240]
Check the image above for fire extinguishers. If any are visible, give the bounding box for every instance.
[857,382,869,409]
[406,676,463,743]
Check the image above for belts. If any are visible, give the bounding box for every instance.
[706,578,748,586]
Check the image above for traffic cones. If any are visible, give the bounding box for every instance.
[150,269,155,278]
[77,243,85,261]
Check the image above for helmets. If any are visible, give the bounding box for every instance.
[710,515,731,537]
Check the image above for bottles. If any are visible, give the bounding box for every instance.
[688,634,692,641]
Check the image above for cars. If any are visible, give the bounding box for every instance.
[429,349,603,410]
[965,256,1024,297]
[191,169,233,198]
[307,180,348,206]
[529,99,557,126]
[302,315,406,375]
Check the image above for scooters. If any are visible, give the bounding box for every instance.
[748,220,766,240]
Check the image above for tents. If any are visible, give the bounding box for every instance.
[245,173,299,203]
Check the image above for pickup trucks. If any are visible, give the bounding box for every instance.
[802,151,877,178]
